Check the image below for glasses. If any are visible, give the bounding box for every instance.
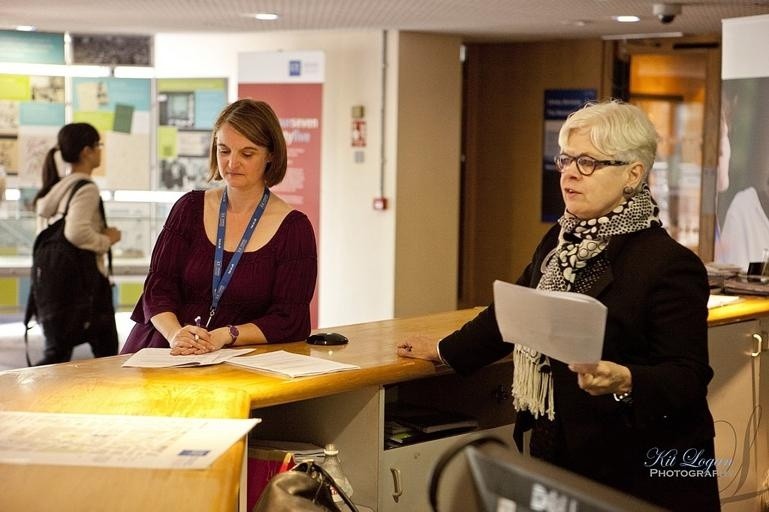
[554,153,630,174]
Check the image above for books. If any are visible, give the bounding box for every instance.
[394,410,479,433]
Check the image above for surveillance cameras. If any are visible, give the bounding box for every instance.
[652,5,683,25]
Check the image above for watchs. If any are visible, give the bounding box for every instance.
[225,324,239,347]
[613,391,631,405]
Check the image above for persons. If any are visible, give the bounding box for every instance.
[27,121,120,363]
[713,89,768,271]
[393,96,721,511]
[120,98,318,355]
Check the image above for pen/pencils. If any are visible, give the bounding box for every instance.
[195,316,201,341]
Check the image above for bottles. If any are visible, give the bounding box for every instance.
[317,443,354,512]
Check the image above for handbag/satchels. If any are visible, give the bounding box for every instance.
[254,461,357,512]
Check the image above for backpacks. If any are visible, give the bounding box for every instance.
[24,180,110,368]
